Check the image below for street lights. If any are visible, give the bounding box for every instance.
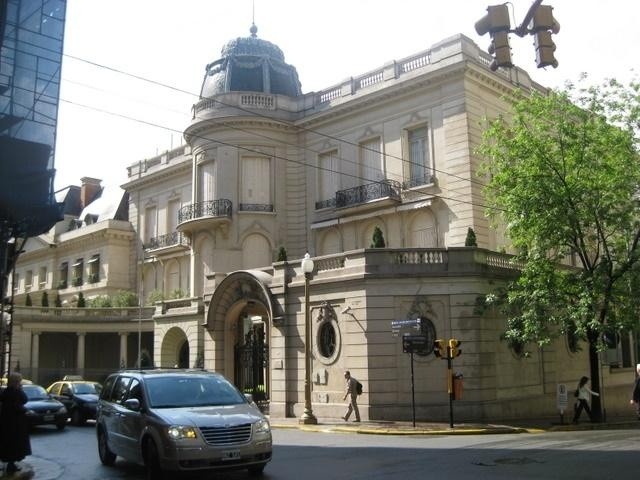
[299,252,317,425]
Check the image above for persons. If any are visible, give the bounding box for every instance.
[0,372,32,473]
[342,370,361,423]
[630,364,640,420]
[572,375,602,423]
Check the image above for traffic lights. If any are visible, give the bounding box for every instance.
[474,4,513,71]
[529,4,560,68]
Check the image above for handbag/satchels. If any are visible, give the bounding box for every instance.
[574,383,580,397]
[351,377,363,395]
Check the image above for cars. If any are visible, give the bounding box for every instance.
[96,367,272,480]
[0,375,104,431]
